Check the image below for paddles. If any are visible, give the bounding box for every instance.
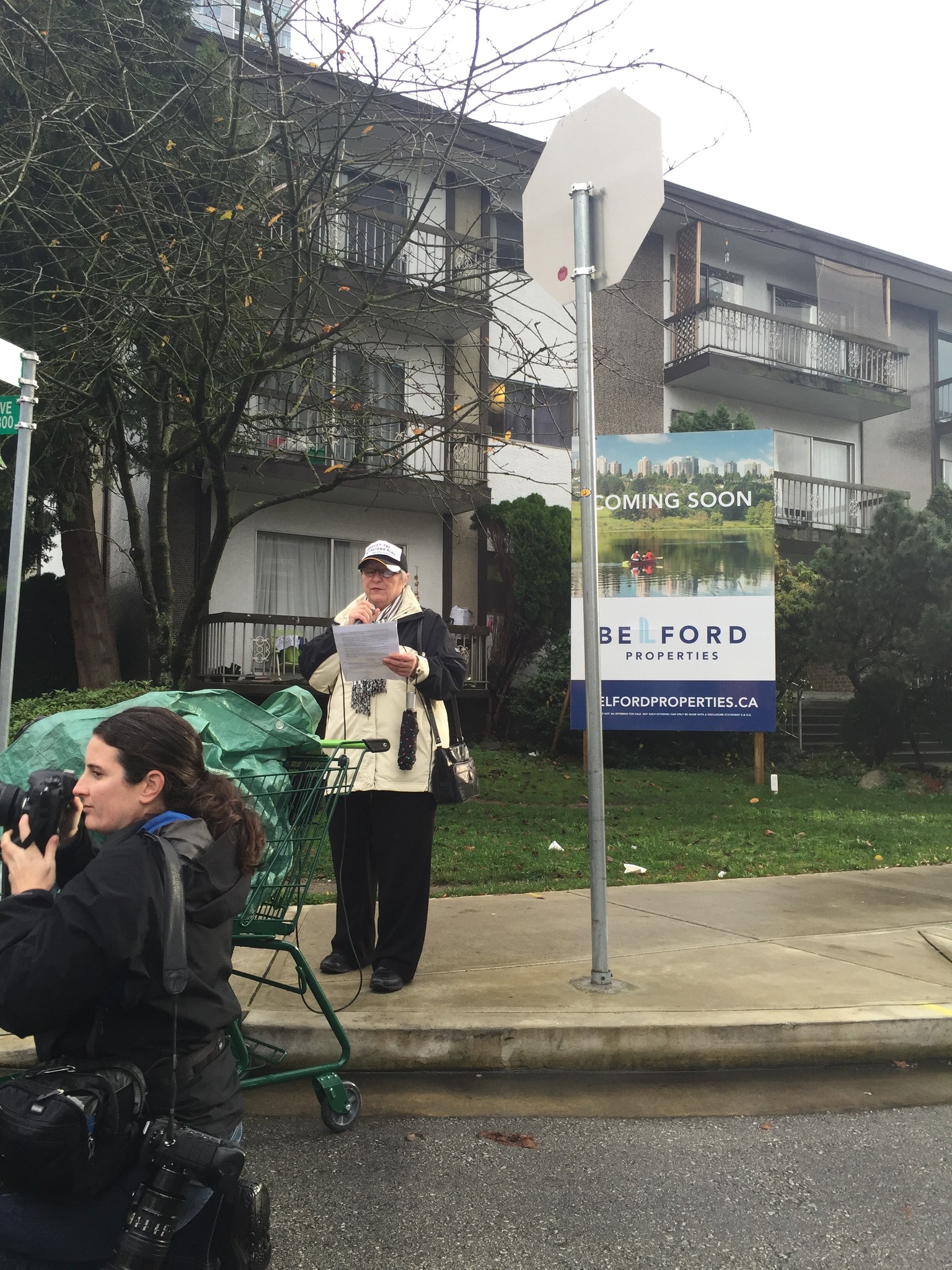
[622,559,632,564]
[654,556,663,560]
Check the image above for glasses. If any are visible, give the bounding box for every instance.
[360,567,396,577]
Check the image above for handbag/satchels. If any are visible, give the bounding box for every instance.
[433,742,480,805]
[0,1058,147,1186]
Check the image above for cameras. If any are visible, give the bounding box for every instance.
[0,766,76,848]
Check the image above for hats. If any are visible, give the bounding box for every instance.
[358,540,408,573]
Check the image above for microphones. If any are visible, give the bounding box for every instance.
[353,602,375,624]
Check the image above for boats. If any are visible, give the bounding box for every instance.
[628,557,656,568]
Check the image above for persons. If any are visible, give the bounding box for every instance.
[0,709,263,1152]
[631,551,640,561]
[302,538,467,990]
[642,553,648,560]
[646,549,653,560]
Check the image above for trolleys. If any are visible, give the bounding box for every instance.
[0,677,392,1134]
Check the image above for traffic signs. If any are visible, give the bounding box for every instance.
[0,393,21,434]
[1,339,25,388]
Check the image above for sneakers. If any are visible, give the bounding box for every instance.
[320,952,357,975]
[369,966,404,991]
